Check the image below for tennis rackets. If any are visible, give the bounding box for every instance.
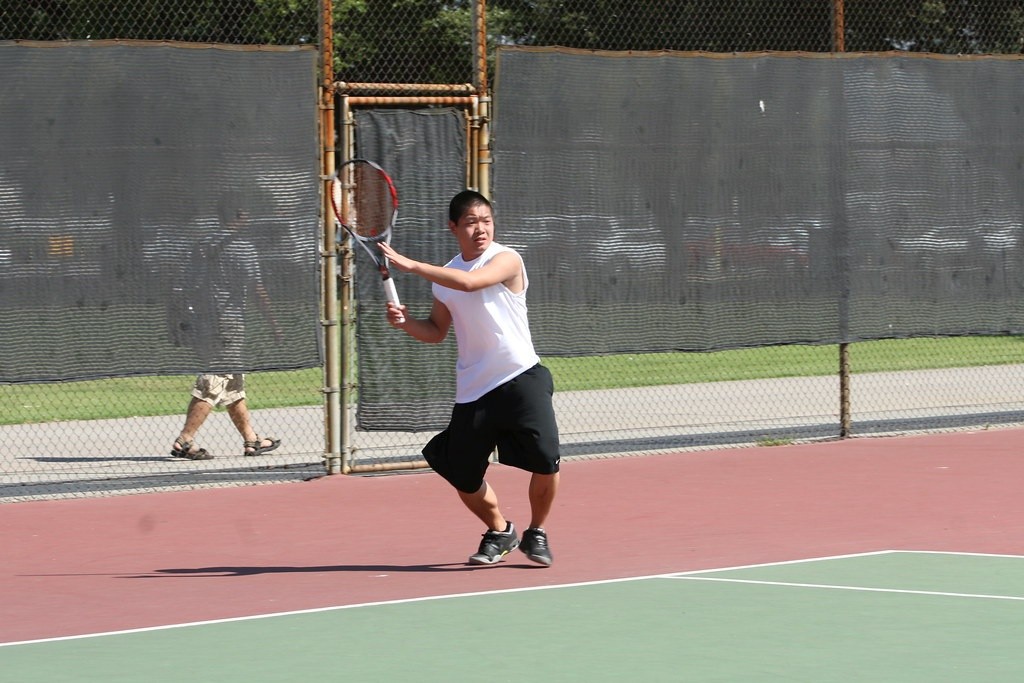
[328,158,406,326]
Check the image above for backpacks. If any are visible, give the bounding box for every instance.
[170,231,242,361]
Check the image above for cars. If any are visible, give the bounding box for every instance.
[0,170,1024,280]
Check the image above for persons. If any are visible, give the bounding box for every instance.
[169,193,281,460]
[377,190,562,566]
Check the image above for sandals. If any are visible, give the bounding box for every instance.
[171,437,214,460]
[243,434,281,456]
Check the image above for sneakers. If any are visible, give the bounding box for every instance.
[518,529,552,566]
[468,521,520,565]
